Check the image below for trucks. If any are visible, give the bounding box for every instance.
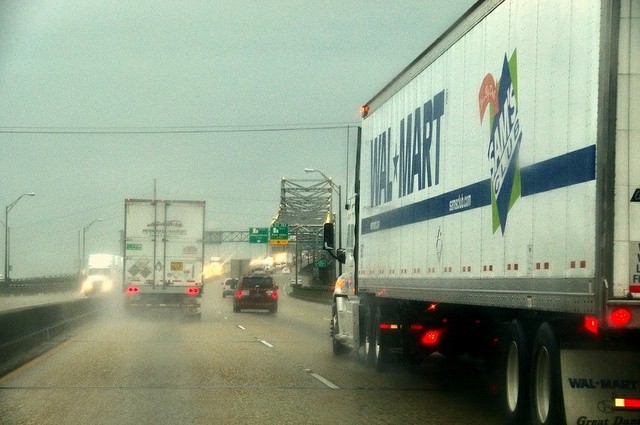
[322,0,640,425]
[124,199,205,319]
[231,259,249,277]
[85,254,123,297]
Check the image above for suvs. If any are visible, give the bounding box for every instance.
[231,275,279,312]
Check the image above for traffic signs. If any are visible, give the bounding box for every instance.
[270,226,288,245]
[249,228,268,243]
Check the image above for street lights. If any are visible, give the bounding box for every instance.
[5,193,35,287]
[305,168,342,276]
[83,219,102,272]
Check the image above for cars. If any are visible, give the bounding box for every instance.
[281,267,290,274]
[290,277,302,285]
[221,278,238,297]
